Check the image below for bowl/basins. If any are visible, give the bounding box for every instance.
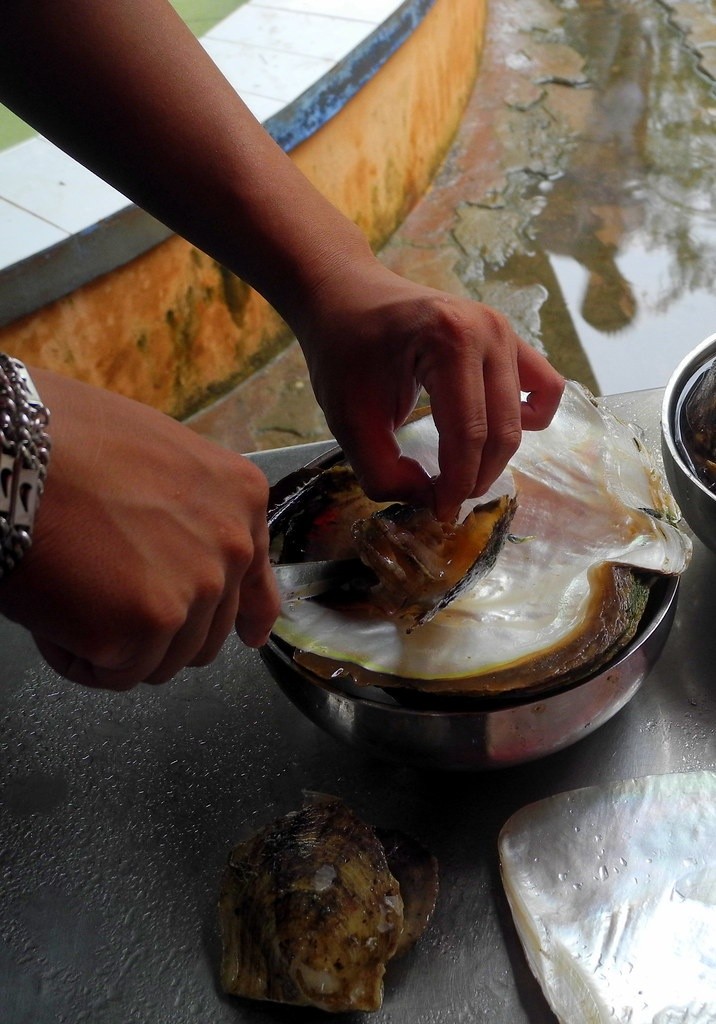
[661,331,716,555]
[257,401,681,774]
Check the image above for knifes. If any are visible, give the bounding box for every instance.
[269,554,377,604]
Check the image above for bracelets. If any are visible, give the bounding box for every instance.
[0,355,50,574]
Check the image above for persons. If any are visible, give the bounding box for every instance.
[1,0,567,692]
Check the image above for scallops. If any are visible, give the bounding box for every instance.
[200,783,442,1013]
[266,456,522,635]
